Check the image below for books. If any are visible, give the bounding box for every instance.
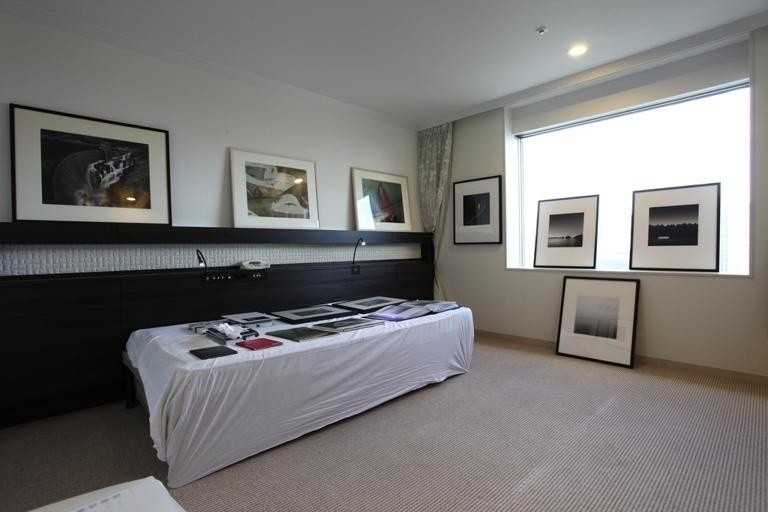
[265,326,339,343]
[312,318,384,333]
[366,299,462,321]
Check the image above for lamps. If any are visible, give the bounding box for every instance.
[351,236,367,275]
[196,249,211,283]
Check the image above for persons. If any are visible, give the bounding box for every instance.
[377,182,394,221]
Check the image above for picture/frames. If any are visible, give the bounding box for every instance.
[453,174,503,246]
[350,167,414,232]
[533,193,599,269]
[9,102,173,231]
[230,147,321,232]
[329,294,409,314]
[629,182,721,273]
[269,302,358,323]
[555,275,640,369]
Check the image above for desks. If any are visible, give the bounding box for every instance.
[26,476,187,512]
[121,296,476,490]
